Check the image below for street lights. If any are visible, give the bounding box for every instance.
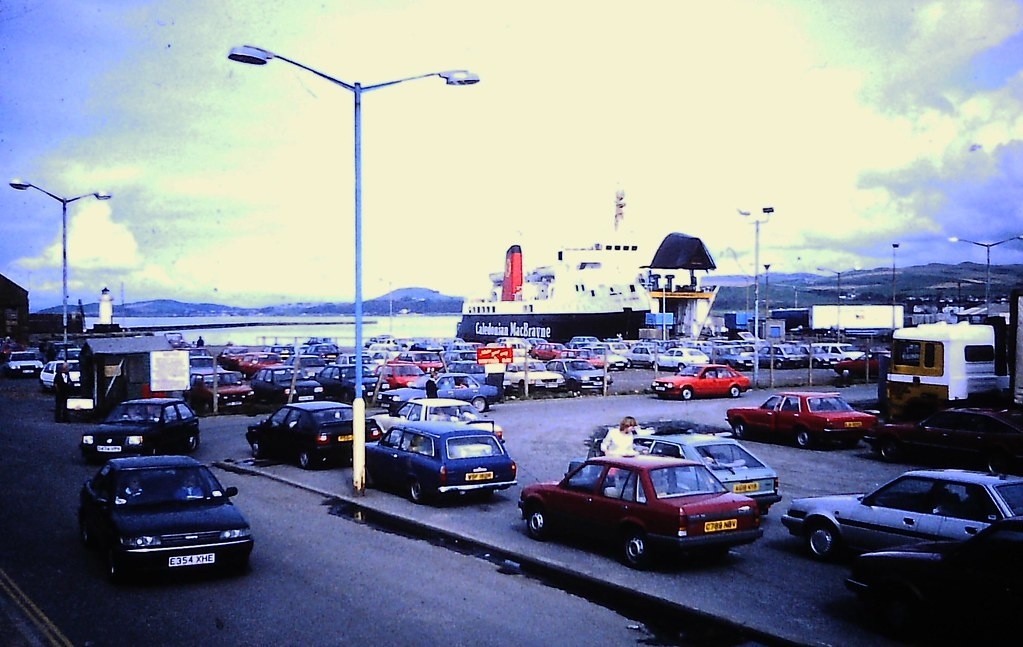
[816,267,863,340]
[734,207,777,385]
[948,235,1023,314]
[8,176,111,341]
[891,243,900,328]
[225,42,479,488]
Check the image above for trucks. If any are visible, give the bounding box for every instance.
[889,322,1009,412]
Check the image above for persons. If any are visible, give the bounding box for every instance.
[53,361,74,422]
[197,336,204,348]
[601,416,640,456]
[99,287,114,324]
[459,382,467,389]
[425,375,439,399]
[441,378,452,391]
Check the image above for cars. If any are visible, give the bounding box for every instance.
[77,452,253,577]
[243,401,379,470]
[364,426,518,504]
[570,435,782,518]
[865,407,1022,476]
[780,467,1023,646]
[518,451,765,568]
[367,398,506,444]
[164,331,877,410]
[725,391,879,449]
[81,398,198,465]
[1,339,83,391]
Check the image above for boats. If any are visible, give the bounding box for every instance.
[454,186,717,344]
